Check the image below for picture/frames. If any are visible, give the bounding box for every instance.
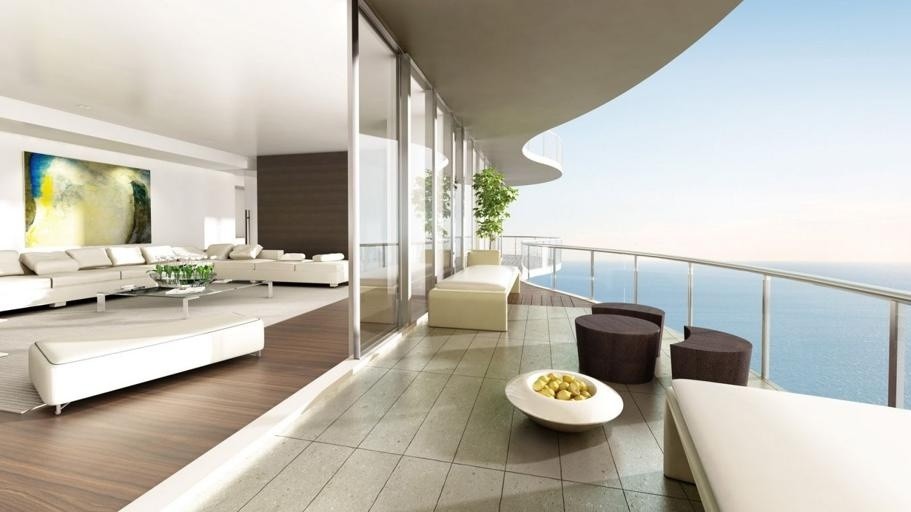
[24,153,151,247]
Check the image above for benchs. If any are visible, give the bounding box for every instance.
[427,264,521,333]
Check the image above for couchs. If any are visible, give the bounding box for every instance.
[0,245,213,312]
[204,244,348,288]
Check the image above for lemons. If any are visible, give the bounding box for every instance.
[528,369,592,401]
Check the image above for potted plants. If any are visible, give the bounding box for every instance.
[149,265,217,289]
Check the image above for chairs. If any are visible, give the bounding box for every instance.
[467,251,501,266]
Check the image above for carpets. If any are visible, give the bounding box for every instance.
[0,286,373,413]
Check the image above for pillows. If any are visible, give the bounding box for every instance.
[229,244,262,260]
[65,247,111,269]
[142,245,177,264]
[173,246,206,260]
[21,251,78,274]
[280,253,306,261]
[206,244,235,259]
[0,251,23,276]
[313,252,343,262]
[107,247,145,267]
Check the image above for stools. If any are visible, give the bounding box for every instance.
[663,379,911,510]
[29,313,265,413]
[592,303,665,355]
[670,325,753,386]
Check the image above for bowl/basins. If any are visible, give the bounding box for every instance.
[505,369,625,431]
